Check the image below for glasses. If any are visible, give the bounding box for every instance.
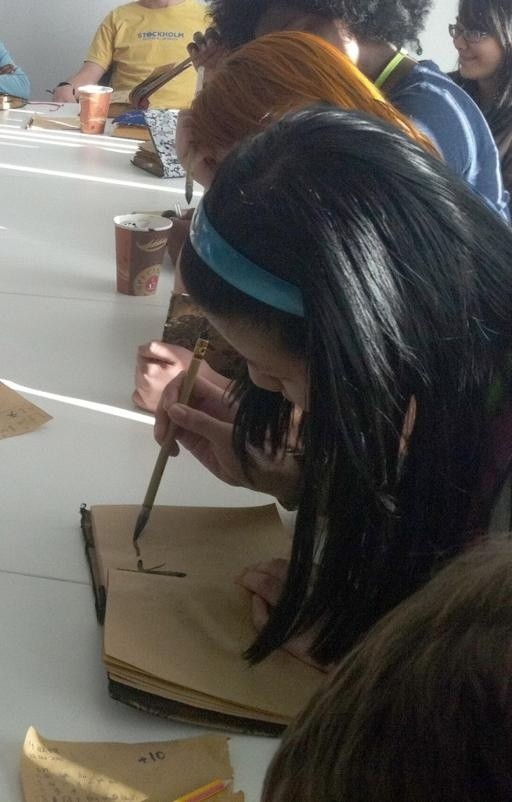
[448,24,491,43]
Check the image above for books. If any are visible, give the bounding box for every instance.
[81,500,326,737]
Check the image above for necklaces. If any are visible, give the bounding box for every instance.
[373,45,409,89]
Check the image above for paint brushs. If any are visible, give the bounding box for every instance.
[133,334,209,543]
[185,68,206,203]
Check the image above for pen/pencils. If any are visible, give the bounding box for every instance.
[25,114,37,130]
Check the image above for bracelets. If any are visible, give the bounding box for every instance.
[46,81,75,96]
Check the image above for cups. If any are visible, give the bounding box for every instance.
[111,211,172,297]
[73,84,115,135]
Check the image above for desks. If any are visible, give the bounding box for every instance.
[1,91,330,801]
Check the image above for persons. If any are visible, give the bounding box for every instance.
[261,535,512,802]
[154,105,512,663]
[0,38,31,97]
[51,0,214,112]
[135,31,428,450]
[186,3,503,211]
[448,1,512,222]
